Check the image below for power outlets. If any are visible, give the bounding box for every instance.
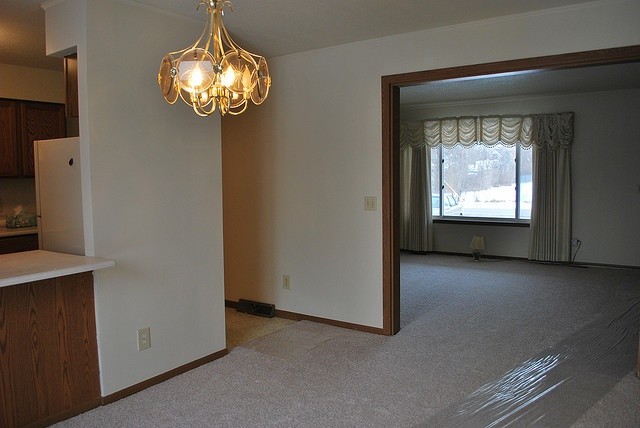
[363,195,379,212]
[281,274,291,288]
[136,325,152,351]
[571,238,582,247]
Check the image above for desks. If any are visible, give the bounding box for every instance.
[0,249,116,428]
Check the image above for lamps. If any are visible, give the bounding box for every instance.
[468,234,486,262]
[157,1,273,118]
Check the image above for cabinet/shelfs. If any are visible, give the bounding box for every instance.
[1,101,66,180]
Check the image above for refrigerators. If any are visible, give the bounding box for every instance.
[33,137,85,256]
